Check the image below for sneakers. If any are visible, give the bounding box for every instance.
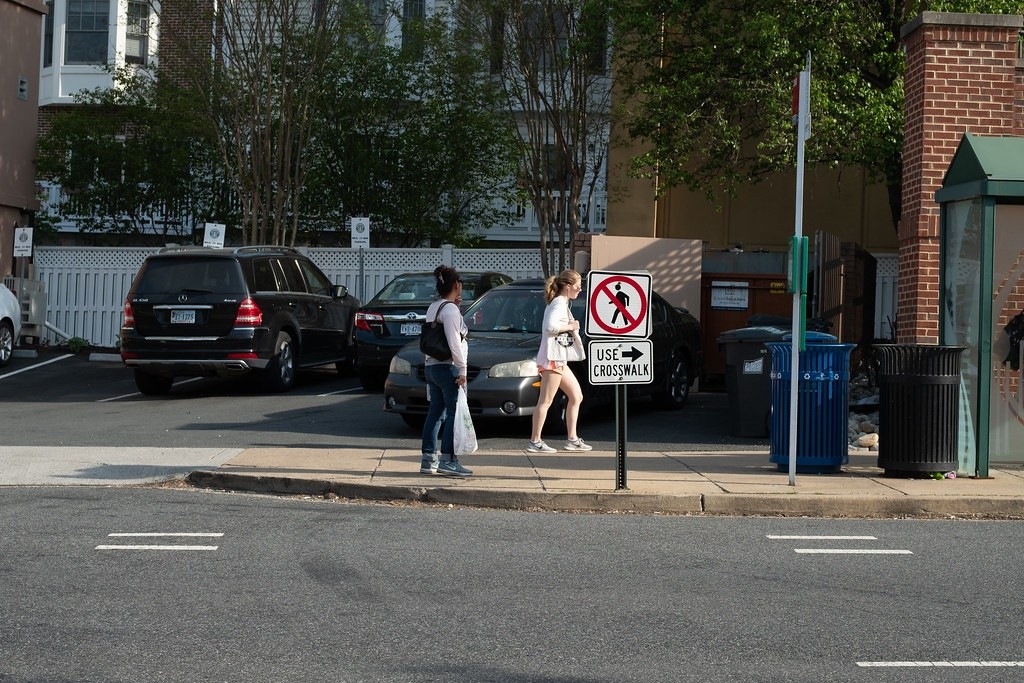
[527,438,557,453]
[437,460,473,476]
[564,437,592,451]
[420,461,439,475]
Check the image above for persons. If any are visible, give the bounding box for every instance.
[420,264,474,477]
[527,269,593,453]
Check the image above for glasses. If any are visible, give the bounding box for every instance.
[454,279,463,284]
[569,283,581,289]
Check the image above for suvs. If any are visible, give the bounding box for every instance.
[120,244,362,397]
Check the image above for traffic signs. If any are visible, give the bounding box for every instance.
[587,340,654,386]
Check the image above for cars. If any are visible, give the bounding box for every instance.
[351,272,515,382]
[0,282,23,368]
[382,279,706,436]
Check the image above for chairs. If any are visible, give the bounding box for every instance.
[209,263,228,287]
[518,303,543,331]
[405,285,428,299]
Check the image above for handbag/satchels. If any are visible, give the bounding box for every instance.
[453,384,478,455]
[546,308,587,361]
[420,301,464,362]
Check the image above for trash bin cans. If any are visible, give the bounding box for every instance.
[746,315,833,334]
[766,331,859,475]
[872,343,969,479]
[716,325,790,437]
